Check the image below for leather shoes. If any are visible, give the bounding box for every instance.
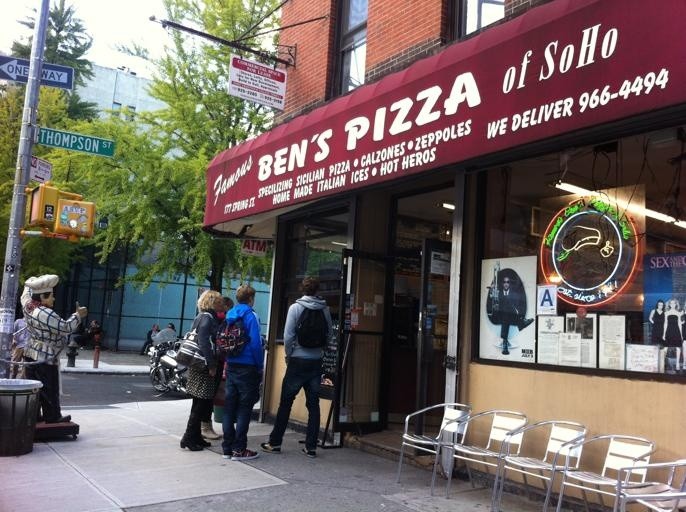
[519,318,534,331]
[502,344,509,355]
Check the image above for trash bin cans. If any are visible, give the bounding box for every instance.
[0,378,44,456]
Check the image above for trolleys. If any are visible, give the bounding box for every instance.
[34,419,80,441]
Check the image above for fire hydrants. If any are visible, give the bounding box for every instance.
[66,340,79,367]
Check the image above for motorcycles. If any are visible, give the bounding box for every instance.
[146,338,193,398]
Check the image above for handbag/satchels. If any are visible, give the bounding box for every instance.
[175,331,213,371]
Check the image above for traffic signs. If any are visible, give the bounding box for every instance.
[0,57,73,91]
[34,126,115,158]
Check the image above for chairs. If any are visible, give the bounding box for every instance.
[615,460,686,512]
[396,402,476,496]
[496,420,591,511]
[446,410,530,512]
[555,435,657,512]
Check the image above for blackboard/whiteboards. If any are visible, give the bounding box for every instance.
[319,320,345,399]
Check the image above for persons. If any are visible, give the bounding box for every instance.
[200,297,233,440]
[141,324,160,355]
[490,276,535,355]
[10,315,27,379]
[180,289,224,451]
[222,285,265,461]
[20,274,88,424]
[168,323,176,336]
[648,299,686,372]
[260,277,332,459]
[80,320,102,349]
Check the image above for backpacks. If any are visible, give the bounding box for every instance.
[215,319,250,357]
[295,308,329,349]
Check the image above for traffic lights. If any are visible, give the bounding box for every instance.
[27,183,58,229]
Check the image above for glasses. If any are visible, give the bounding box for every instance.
[503,281,510,283]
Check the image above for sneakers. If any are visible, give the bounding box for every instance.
[260,442,281,454]
[301,447,317,458]
[223,449,259,460]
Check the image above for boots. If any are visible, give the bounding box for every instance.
[181,420,221,451]
